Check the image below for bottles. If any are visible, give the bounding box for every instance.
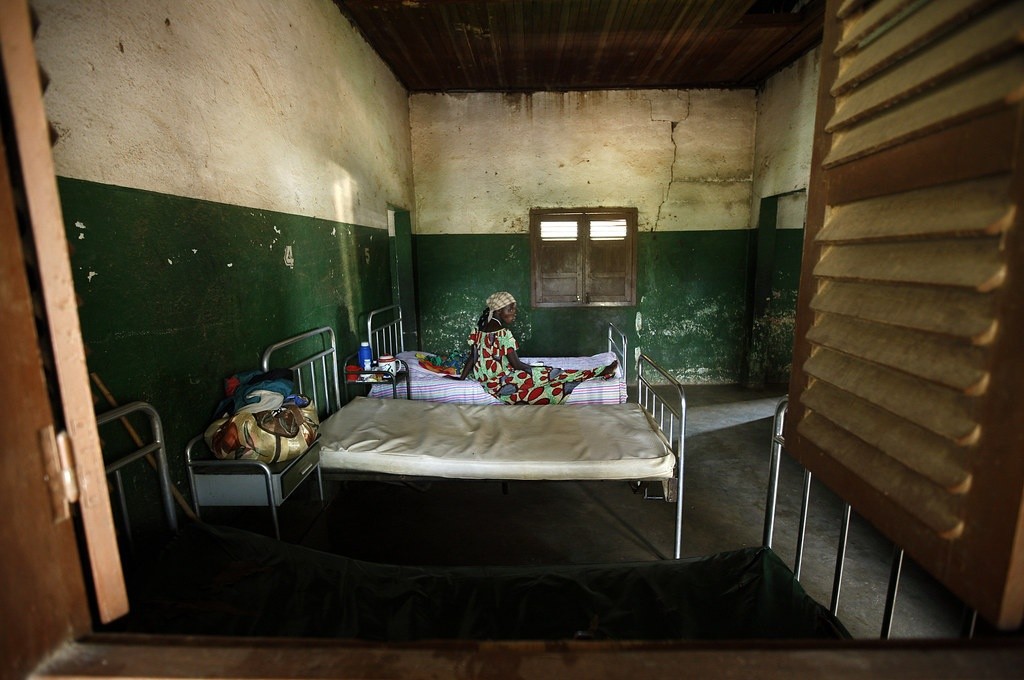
[371,361,378,371]
[364,359,371,372]
[359,342,372,371]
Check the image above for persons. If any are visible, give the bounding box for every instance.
[442,292,618,407]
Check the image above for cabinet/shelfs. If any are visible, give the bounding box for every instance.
[344,351,411,402]
[186,433,326,553]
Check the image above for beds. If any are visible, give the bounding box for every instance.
[368,304,629,405]
[93,395,980,641]
[263,327,686,559]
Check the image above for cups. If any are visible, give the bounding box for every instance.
[346,364,363,380]
[378,354,401,378]
